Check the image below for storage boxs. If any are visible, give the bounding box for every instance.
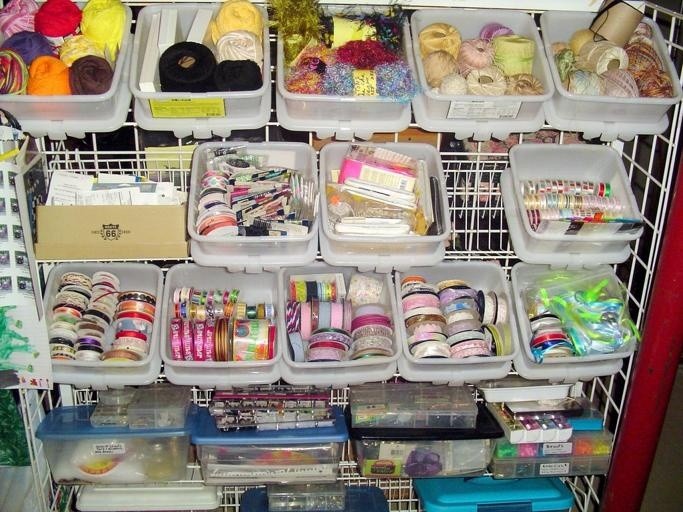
[0,0,682,512]
